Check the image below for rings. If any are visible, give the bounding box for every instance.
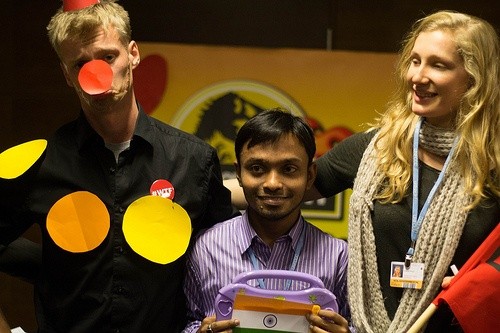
[207,324,212,329]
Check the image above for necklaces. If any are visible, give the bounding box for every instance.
[247,215,307,291]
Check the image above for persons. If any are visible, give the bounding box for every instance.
[0,0,222,333]
[182,107,349,333]
[223,11,500,333]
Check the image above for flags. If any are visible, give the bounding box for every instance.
[433,222,500,333]
